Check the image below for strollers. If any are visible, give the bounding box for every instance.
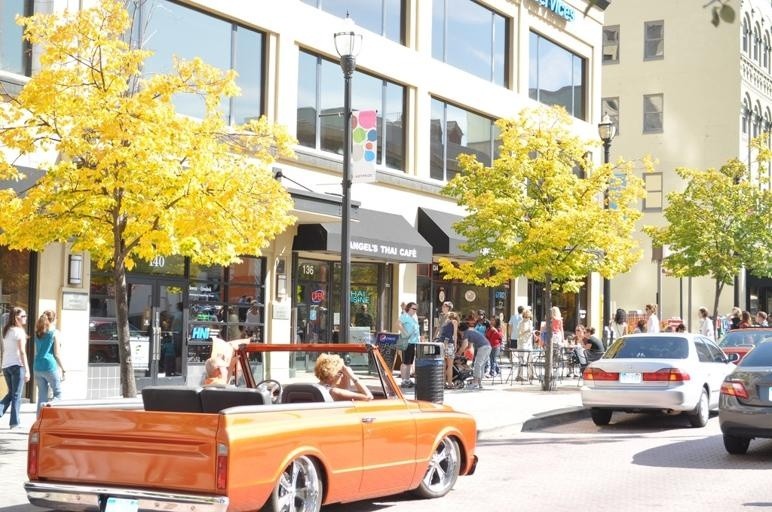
[439,349,472,389]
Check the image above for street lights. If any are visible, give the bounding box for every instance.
[330,10,363,364]
[597,110,617,349]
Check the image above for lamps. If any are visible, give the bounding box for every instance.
[68,254,82,284]
[276,274,287,301]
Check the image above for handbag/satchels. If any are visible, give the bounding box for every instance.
[395,338,408,352]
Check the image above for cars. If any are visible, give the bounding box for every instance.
[87,297,329,363]
[720,337,772,455]
[579,331,741,428]
[711,326,772,368]
[20,338,484,512]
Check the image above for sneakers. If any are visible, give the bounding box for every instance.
[401,379,414,388]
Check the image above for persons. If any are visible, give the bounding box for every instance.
[697,306,770,344]
[564,308,629,378]
[633,320,647,333]
[1,307,29,430]
[352,303,375,329]
[309,352,374,401]
[434,301,503,391]
[396,302,419,387]
[35,308,66,420]
[646,304,660,332]
[661,324,687,333]
[200,352,235,387]
[154,291,262,377]
[508,306,564,382]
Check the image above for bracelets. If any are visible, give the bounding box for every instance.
[353,379,359,383]
[62,370,66,373]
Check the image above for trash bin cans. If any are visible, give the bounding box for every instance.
[415,342,444,404]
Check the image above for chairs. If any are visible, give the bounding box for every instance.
[490,343,604,386]
[141,383,334,414]
